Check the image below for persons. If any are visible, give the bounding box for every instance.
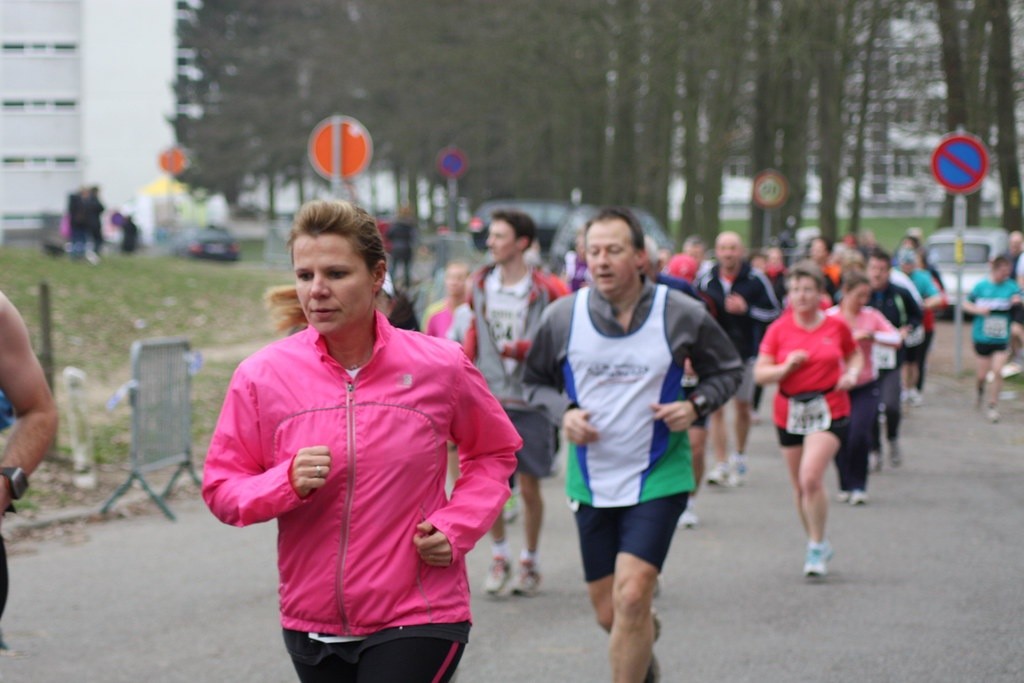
[201,198,523,683]
[961,230,1024,421]
[0,290,58,618]
[517,209,746,683]
[378,208,947,593]
[68,185,103,258]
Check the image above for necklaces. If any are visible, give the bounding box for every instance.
[349,343,373,370]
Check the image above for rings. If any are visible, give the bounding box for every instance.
[316,465,321,477]
[428,555,434,560]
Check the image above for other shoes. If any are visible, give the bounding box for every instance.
[987,407,1000,423]
[642,609,660,683]
[501,491,517,521]
[910,387,925,407]
[805,540,833,574]
[731,463,746,486]
[899,390,908,411]
[851,488,866,504]
[869,449,882,472]
[837,489,848,501]
[679,503,697,528]
[889,440,902,466]
[512,560,540,596]
[707,463,729,485]
[485,556,511,594]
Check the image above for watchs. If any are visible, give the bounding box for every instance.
[691,393,710,416]
[0,467,28,500]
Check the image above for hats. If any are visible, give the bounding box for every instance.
[898,248,916,266]
[670,253,698,282]
[790,259,823,285]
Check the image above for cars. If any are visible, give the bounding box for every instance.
[929,225,1007,305]
[468,198,570,249]
[175,227,241,262]
[548,211,670,277]
